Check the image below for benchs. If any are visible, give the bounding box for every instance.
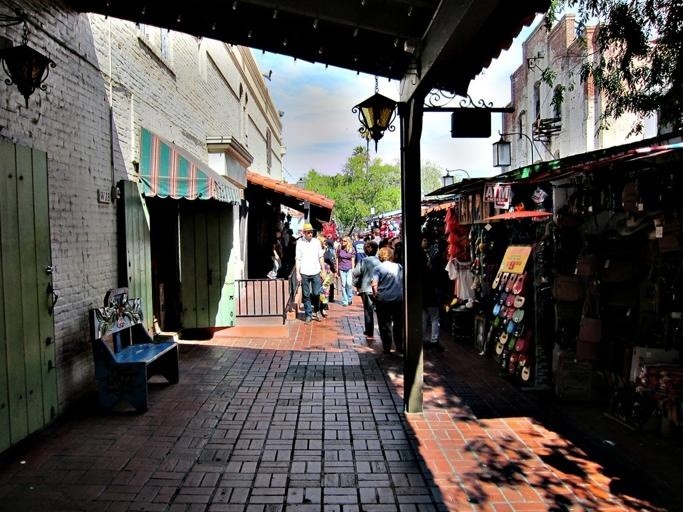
[89,287,179,416]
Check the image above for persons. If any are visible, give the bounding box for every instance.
[294,224,323,322]
[269,218,294,279]
[319,224,446,358]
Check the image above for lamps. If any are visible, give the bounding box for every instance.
[0,12,56,109]
[442,168,470,187]
[352,72,401,153]
[493,130,533,167]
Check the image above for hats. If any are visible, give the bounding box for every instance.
[298,223,315,232]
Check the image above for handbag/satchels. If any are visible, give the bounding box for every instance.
[351,272,363,288]
[554,243,604,403]
